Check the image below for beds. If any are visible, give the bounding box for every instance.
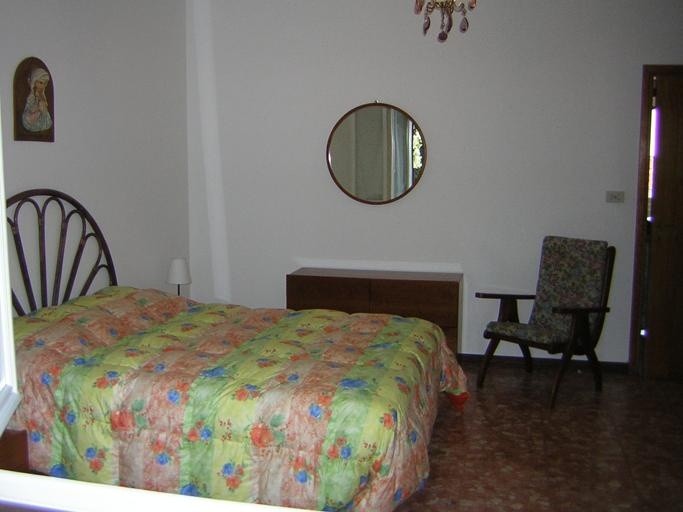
[4,186,469,512]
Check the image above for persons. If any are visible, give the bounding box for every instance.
[19,67,53,133]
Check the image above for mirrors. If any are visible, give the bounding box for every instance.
[324,99,429,209]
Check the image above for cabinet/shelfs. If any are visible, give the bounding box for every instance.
[281,259,462,359]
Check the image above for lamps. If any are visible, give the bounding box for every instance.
[163,256,191,299]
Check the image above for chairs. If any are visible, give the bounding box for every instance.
[472,234,620,413]
